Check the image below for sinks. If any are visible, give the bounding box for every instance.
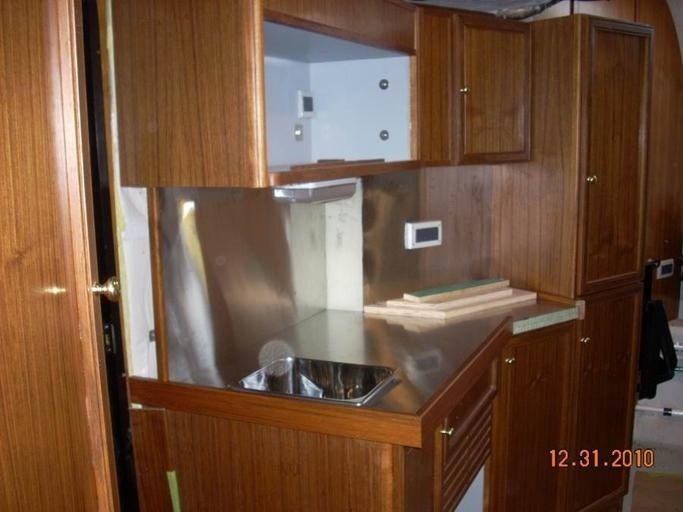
[227,355,402,405]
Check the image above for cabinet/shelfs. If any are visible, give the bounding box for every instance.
[409,362,502,509]
[501,317,573,509]
[505,27,651,293]
[559,281,640,511]
[457,24,536,159]
[124,2,449,188]
